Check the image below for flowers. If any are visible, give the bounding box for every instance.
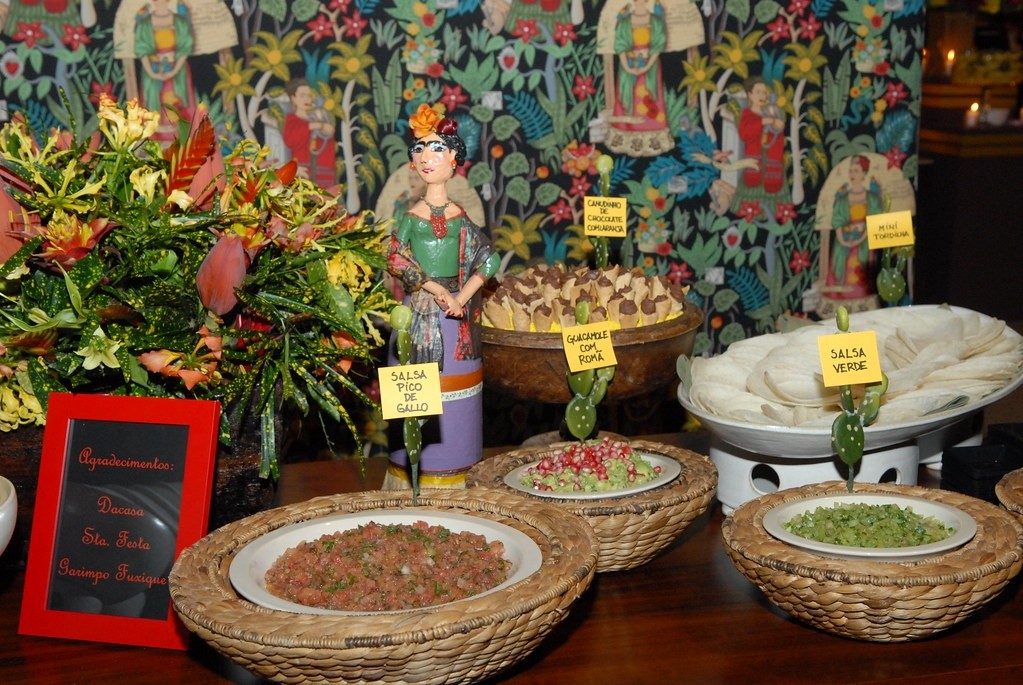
[0,76,424,510]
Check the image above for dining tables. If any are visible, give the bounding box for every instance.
[0,431,1023,685]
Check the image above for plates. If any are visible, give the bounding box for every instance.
[763,491,980,563]
[501,444,683,501]
[229,506,544,619]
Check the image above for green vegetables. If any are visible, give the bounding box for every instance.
[315,520,489,598]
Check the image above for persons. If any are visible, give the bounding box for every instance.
[386,100,501,473]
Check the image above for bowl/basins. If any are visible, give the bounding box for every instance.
[478,300,707,406]
[0,474,19,556]
[677,303,1023,461]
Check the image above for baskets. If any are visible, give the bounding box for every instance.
[995,468,1023,523]
[721,481,1023,643]
[465,440,717,573]
[168,486,598,685]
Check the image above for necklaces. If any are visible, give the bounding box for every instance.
[421,194,451,237]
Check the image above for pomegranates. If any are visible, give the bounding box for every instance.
[523,435,661,493]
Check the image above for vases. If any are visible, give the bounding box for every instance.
[207,449,278,533]
[0,411,47,570]
